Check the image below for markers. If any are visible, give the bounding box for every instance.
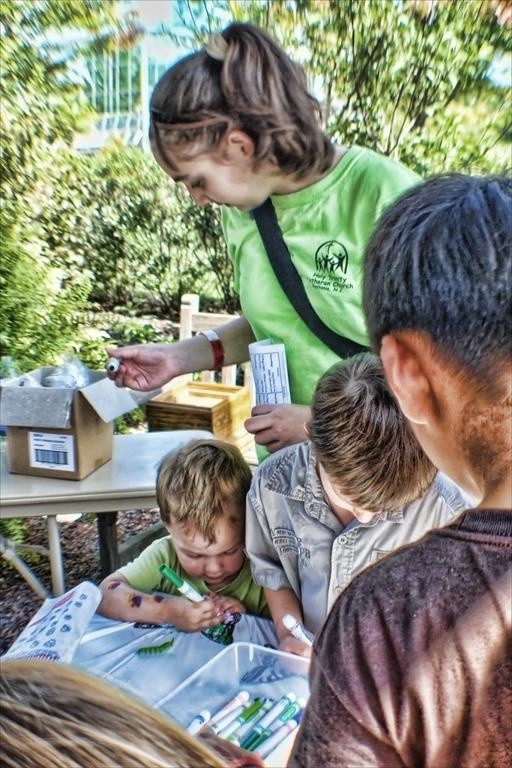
[107,357,123,374]
[185,690,307,759]
[282,614,315,646]
[159,564,205,603]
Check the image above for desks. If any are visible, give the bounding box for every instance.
[76,613,317,760]
[0,430,216,599]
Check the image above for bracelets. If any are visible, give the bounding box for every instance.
[199,328,225,370]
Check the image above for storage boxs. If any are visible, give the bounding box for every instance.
[144,380,255,455]
[0,367,140,480]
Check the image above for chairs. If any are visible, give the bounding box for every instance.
[179,294,256,408]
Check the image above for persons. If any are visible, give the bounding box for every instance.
[0,659,265,768]
[105,23,425,465]
[94,440,273,632]
[285,172,512,767]
[243,353,474,677]
